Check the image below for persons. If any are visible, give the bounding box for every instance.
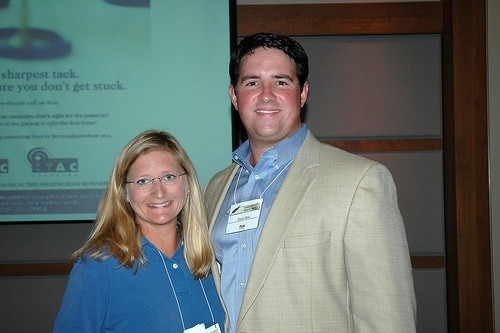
[53,128,229,333]
[196,33,419,333]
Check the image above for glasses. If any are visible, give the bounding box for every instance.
[125,173,188,190]
